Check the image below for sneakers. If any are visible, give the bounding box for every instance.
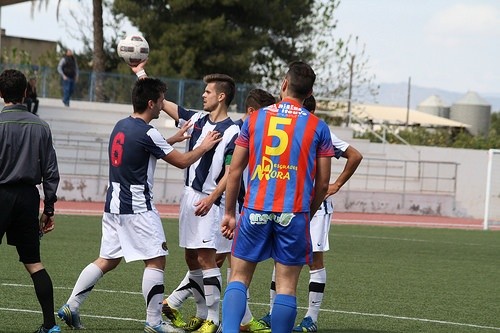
[191,320,222,333]
[292,316,318,333]
[35,324,63,333]
[257,312,272,329]
[240,316,271,333]
[57,304,86,330]
[144,319,185,333]
[161,299,187,329]
[181,316,206,333]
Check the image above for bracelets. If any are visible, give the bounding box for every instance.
[43,210,54,228]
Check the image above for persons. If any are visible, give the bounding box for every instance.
[0,69,61,333]
[127,57,362,333]
[57,49,79,107]
[57,78,223,333]
[23,78,40,116]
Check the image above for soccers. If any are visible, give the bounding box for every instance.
[117,33,150,66]
